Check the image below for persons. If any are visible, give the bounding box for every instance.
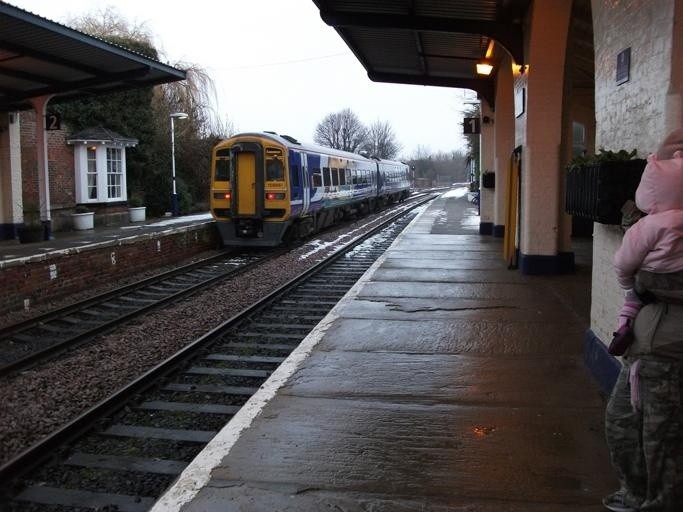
[601,129,682,512]
[609,151,682,358]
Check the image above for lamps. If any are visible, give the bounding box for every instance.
[476,33,524,76]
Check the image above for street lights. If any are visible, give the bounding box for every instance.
[169,112,190,216]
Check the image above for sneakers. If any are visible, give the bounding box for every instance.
[608,323,633,356]
[601,489,642,512]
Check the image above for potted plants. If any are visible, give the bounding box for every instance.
[70,206,95,230]
[467,181,479,203]
[17,200,47,244]
[566,147,647,223]
[127,196,146,222]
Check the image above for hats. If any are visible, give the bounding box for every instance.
[655,127,682,160]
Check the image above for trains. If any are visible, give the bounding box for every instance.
[209,130,410,246]
